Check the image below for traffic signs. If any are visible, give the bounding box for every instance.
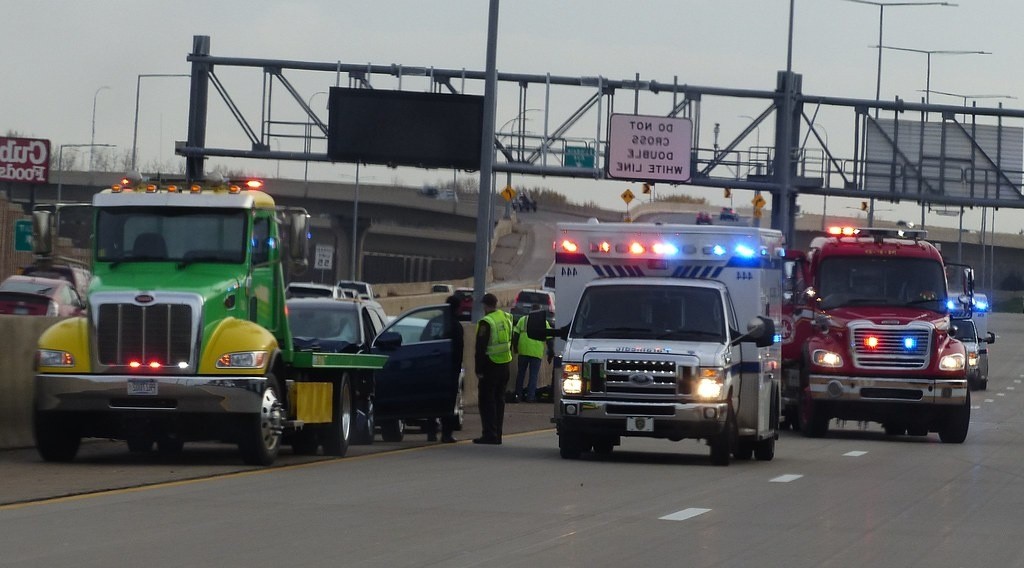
[563,147,596,167]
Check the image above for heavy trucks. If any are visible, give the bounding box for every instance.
[28,175,391,468]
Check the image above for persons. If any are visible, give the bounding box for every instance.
[513,306,553,402]
[474,294,509,447]
[420,297,469,443]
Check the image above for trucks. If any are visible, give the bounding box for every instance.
[549,210,785,466]
[948,291,997,391]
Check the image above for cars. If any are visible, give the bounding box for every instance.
[511,270,555,333]
[284,277,375,301]
[430,280,489,322]
[0,255,92,315]
[379,309,465,430]
[511,192,539,212]
[284,297,454,442]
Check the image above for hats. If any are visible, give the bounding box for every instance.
[481,294,498,305]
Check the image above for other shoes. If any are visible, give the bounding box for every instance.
[427,431,459,444]
[472,436,503,445]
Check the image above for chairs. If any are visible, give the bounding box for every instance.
[134,233,169,259]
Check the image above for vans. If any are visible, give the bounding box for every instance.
[776,224,976,447]
